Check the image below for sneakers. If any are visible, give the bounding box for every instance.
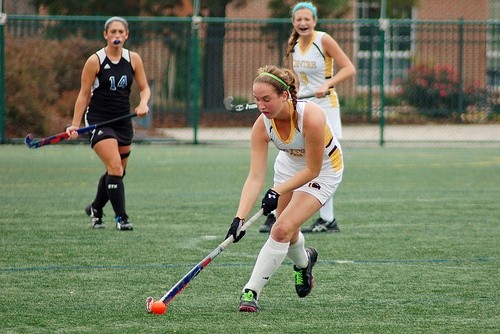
[293,247,318,298]
[115,216,133,230]
[238,290,261,313]
[259,212,276,233]
[300,217,341,233]
[85,203,105,229]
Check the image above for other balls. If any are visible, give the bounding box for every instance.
[151,301,166,315]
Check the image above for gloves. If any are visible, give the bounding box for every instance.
[262,188,280,216]
[225,216,247,242]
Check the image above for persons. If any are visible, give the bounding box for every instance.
[66,17,151,231]
[259,2,356,234]
[226,66,344,312]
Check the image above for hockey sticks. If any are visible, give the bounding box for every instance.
[25,109,150,150]
[145,208,264,315]
[223,90,331,112]
